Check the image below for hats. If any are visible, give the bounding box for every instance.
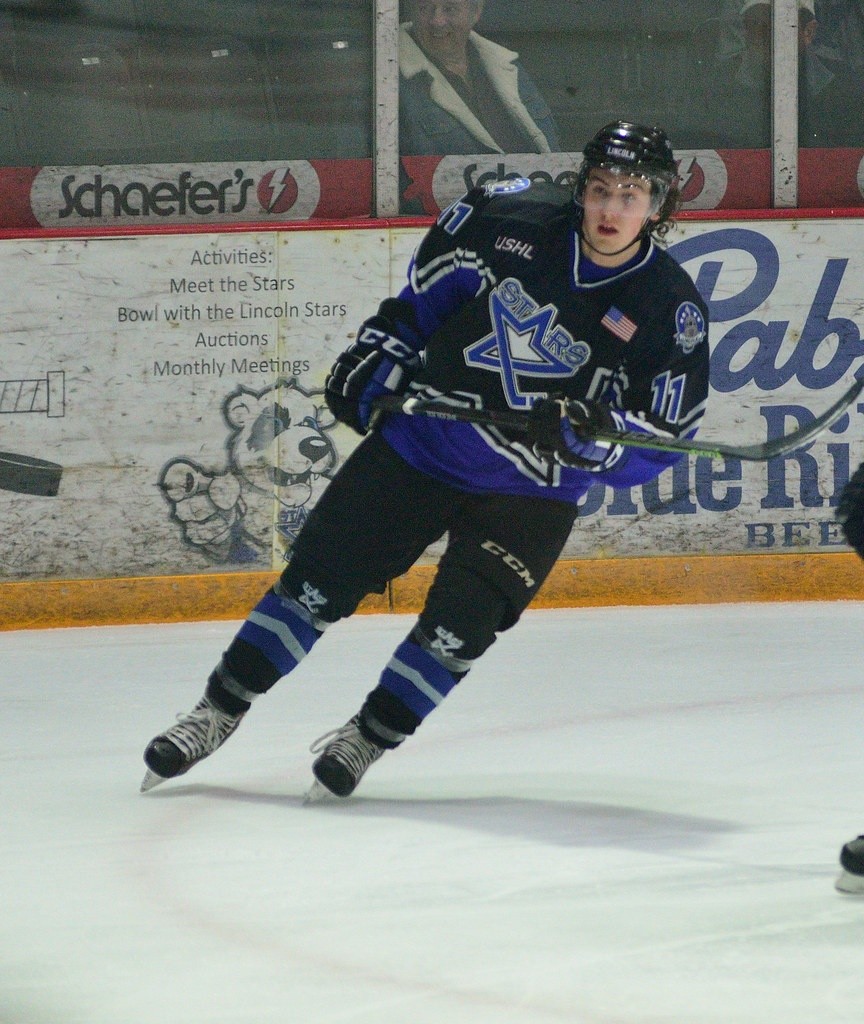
[740,0,815,17]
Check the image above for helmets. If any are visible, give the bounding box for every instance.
[573,120,680,224]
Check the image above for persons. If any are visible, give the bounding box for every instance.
[139,115,713,807]
[393,3,563,158]
[830,464,864,889]
[714,0,864,149]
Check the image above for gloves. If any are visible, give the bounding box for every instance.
[325,297,432,436]
[532,393,626,472]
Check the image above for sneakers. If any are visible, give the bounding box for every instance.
[140,693,245,793]
[835,835,864,894]
[308,715,386,802]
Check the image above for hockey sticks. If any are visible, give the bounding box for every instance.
[373,370,864,465]
